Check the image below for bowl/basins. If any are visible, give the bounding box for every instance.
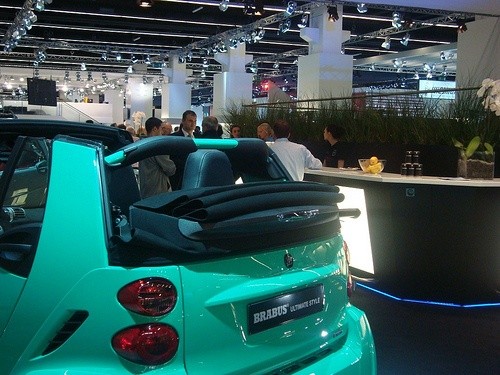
[358,159,387,173]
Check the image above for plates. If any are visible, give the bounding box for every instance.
[340,167,360,171]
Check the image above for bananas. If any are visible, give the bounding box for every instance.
[365,162,381,174]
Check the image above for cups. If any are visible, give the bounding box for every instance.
[84,98,89,103]
[338,159,344,168]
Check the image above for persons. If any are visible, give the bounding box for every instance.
[322,125,348,167]
[257,123,274,142]
[200,116,221,137]
[229,124,243,138]
[137,116,176,200]
[265,120,322,185]
[169,110,203,138]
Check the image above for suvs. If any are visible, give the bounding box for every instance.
[0,115,377,375]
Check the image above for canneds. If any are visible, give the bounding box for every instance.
[400,151,423,176]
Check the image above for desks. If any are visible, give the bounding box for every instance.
[304,166,500,282]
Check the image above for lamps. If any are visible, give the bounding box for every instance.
[0,0,467,102]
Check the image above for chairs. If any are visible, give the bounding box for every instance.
[181,148,235,191]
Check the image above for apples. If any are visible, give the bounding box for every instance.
[369,156,378,165]
